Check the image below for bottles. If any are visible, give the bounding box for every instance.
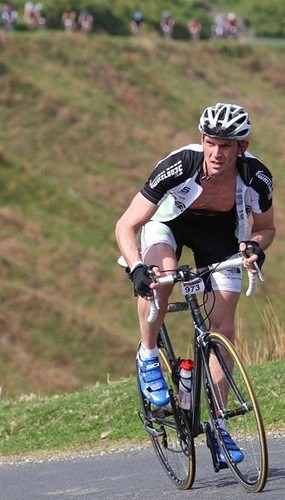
[176,359,196,412]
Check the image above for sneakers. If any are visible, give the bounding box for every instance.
[210,426,244,463]
[135,347,170,407]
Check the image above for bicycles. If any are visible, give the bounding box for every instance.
[0,13,241,42]
[135,242,270,492]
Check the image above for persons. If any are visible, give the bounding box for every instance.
[159,10,175,39]
[188,14,203,42]
[127,10,145,36]
[211,12,238,40]
[114,102,276,464]
[61,4,76,31]
[79,8,94,32]
[3,2,19,31]
[23,2,47,30]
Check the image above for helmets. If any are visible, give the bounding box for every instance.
[197,102,252,141]
